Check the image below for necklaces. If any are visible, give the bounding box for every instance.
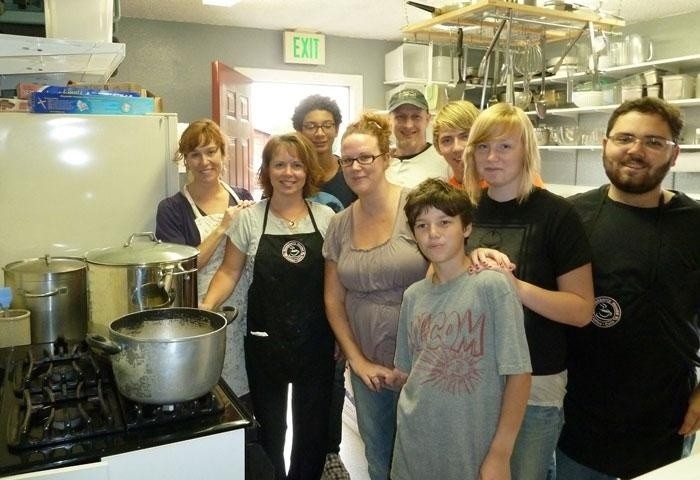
[266,199,307,228]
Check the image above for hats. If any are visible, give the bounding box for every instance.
[388,89,428,114]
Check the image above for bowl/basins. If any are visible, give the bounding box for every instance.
[549,125,583,146]
[532,123,550,146]
[572,91,607,107]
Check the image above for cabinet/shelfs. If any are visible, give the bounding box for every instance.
[380,52,700,154]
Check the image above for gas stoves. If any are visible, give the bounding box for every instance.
[0,335,251,479]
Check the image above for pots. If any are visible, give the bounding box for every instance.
[406,0,483,27]
[84,231,200,355]
[83,304,239,406]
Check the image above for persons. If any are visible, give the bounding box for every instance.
[460,103,595,478]
[262,95,362,214]
[388,177,533,478]
[198,131,344,478]
[155,118,258,443]
[322,111,431,479]
[556,97,700,480]
[383,87,453,191]
[433,101,544,193]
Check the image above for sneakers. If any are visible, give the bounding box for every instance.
[324,453,350,480]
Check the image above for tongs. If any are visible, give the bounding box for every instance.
[478,8,514,85]
[548,20,602,91]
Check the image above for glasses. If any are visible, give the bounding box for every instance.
[338,152,385,167]
[302,122,336,130]
[609,135,676,151]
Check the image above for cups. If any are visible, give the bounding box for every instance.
[610,41,623,70]
[0,309,33,349]
[624,33,654,68]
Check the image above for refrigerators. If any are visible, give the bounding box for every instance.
[0,112,179,284]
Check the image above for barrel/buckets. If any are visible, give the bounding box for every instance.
[82,225,205,369]
